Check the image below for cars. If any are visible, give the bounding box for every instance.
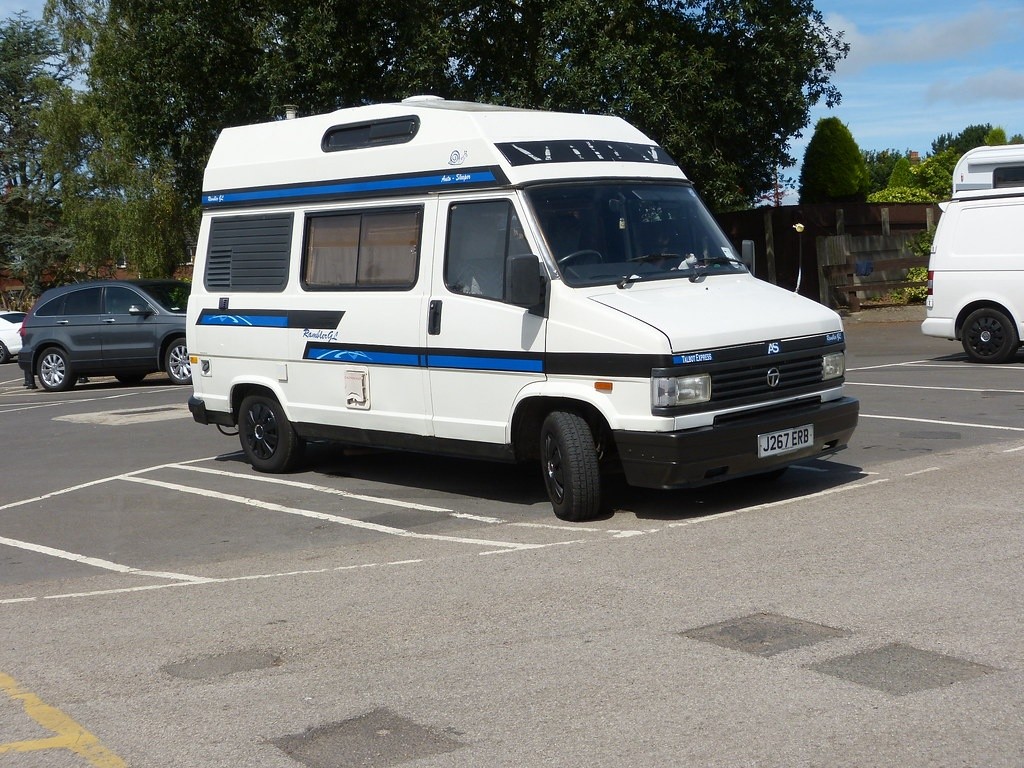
[0,311,28,363]
[18,279,191,392]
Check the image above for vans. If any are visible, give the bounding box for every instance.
[921,144,1024,360]
[184,92,859,522]
